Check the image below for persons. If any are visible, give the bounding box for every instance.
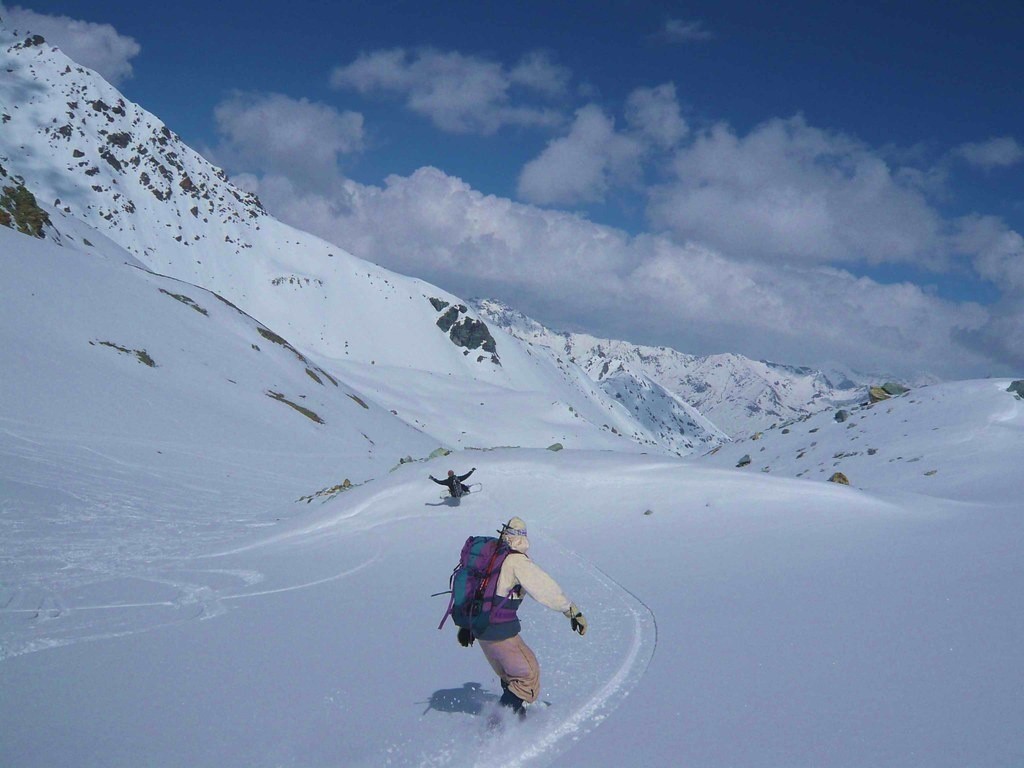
[429,467,476,497]
[458,516,587,732]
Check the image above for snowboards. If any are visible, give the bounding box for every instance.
[520,695,554,717]
[435,480,486,500]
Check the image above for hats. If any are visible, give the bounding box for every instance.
[502,517,529,554]
[448,470,454,476]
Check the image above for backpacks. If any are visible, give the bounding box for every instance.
[450,536,530,638]
[448,476,463,497]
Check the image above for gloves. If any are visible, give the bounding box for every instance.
[457,627,475,647]
[472,468,476,471]
[563,603,586,636]
[429,475,433,479]
